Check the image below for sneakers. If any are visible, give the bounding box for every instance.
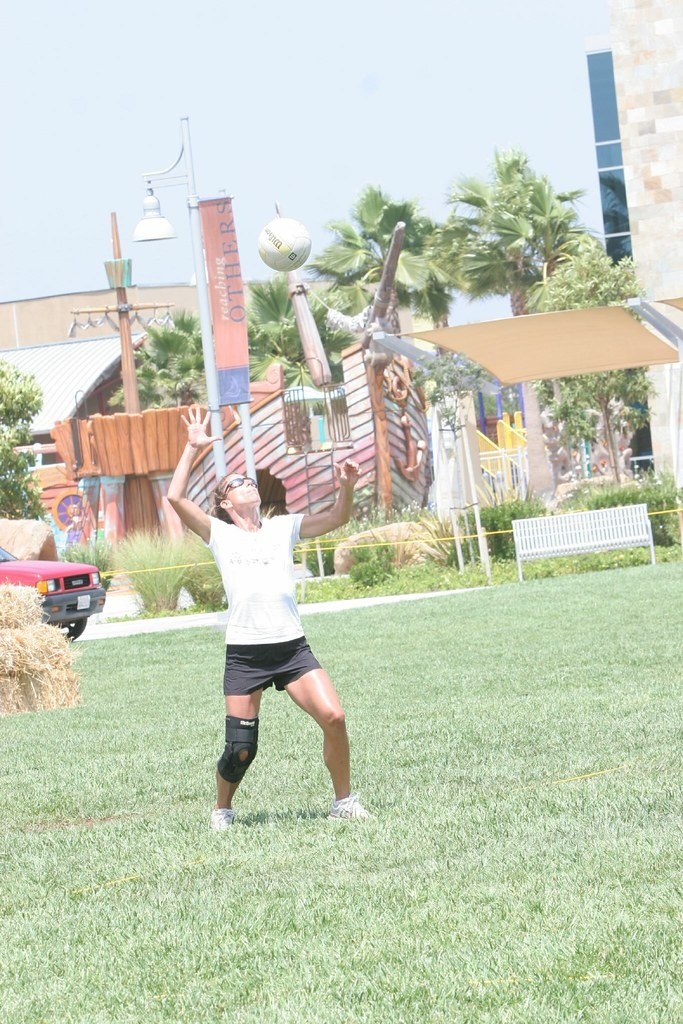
[211,808,235,832]
[328,795,374,822]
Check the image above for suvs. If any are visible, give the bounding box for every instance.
[0,546,106,643]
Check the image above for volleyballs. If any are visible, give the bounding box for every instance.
[256,216,314,274]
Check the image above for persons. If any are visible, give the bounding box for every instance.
[167,404,371,827]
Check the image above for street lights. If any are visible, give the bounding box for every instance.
[131,114,227,489]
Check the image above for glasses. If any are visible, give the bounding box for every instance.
[223,477,258,498]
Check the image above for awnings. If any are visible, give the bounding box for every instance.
[402,305,681,384]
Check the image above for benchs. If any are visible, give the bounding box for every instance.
[512,504,657,586]
